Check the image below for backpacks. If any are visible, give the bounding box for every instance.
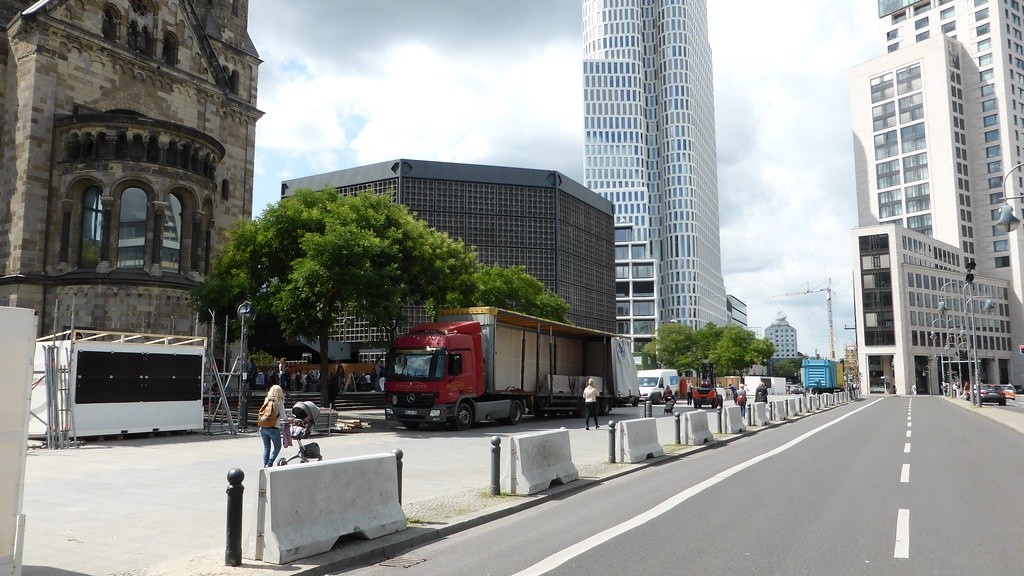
[258,397,280,428]
[737,393,745,405]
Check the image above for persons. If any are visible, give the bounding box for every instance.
[912,383,917,398]
[583,379,599,430]
[245,356,385,394]
[849,382,859,398]
[687,380,695,406]
[290,416,310,437]
[940,381,983,397]
[715,381,768,419]
[259,385,294,468]
[662,385,672,414]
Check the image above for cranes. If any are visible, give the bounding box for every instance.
[773,277,836,360]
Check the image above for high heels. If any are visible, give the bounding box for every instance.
[596,425,599,429]
[586,426,590,430]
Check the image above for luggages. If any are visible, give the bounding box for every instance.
[664,399,674,414]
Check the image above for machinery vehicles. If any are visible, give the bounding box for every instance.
[691,361,723,409]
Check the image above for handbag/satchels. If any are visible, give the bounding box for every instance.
[665,395,674,402]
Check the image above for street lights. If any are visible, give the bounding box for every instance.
[237,301,255,428]
[930,281,996,407]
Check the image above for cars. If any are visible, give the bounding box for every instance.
[1001,385,1016,400]
[974,384,1006,406]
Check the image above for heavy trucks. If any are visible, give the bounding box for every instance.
[379,302,624,431]
[611,338,640,407]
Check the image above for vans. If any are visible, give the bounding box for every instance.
[637,368,680,404]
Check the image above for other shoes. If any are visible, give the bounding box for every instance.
[265,464,270,468]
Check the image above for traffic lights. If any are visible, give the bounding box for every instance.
[1020,345,1024,354]
